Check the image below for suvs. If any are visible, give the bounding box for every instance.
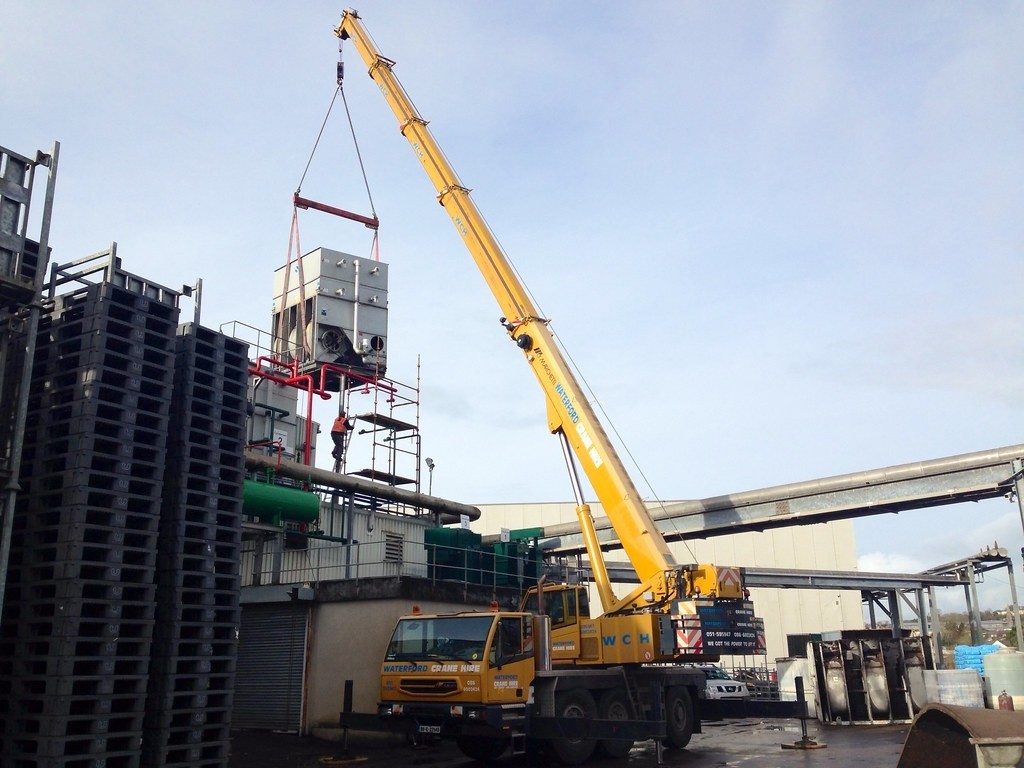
[683,664,751,702]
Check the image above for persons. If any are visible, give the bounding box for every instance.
[331,410,354,461]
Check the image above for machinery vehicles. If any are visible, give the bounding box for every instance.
[248,9,768,768]
[613,211,630,232]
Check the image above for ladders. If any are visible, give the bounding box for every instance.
[323,414,358,503]
